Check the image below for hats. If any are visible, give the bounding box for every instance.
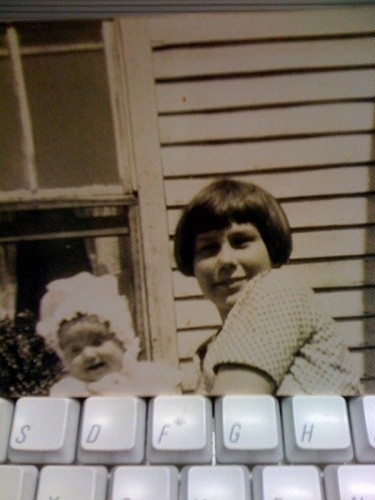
[34,268,137,373]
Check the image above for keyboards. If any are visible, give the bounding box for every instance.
[0,396,375,499]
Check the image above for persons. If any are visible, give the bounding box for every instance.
[173,178,365,397]
[35,272,184,397]
